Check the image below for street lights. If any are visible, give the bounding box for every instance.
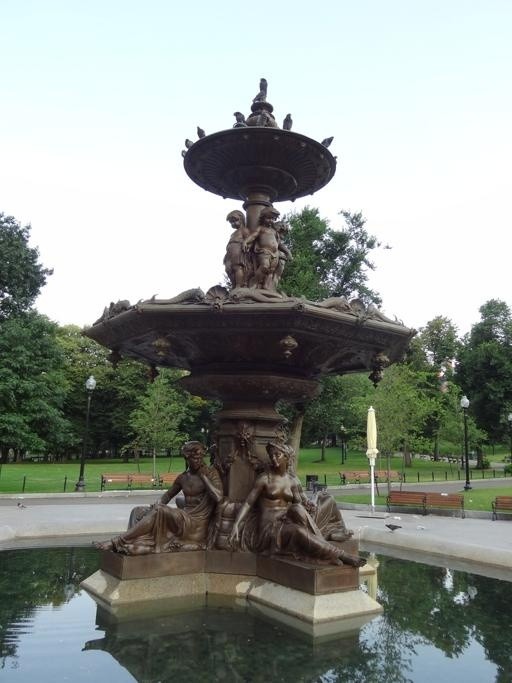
[508,413,512,474]
[75,374,98,494]
[205,429,209,454]
[200,427,205,444]
[459,394,474,490]
[338,425,346,467]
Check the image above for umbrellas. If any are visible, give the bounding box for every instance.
[364,553,379,601]
[366,406,380,512]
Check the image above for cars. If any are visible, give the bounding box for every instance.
[502,455,512,464]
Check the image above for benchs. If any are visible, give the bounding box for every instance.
[337,468,406,486]
[384,489,425,513]
[491,494,512,520]
[421,491,466,520]
[97,472,187,495]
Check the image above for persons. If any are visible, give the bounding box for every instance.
[223,210,252,288]
[272,222,294,289]
[242,207,280,290]
[92,441,224,555]
[228,441,367,567]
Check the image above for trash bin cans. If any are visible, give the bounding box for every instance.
[313,484,327,494]
[306,475,318,491]
